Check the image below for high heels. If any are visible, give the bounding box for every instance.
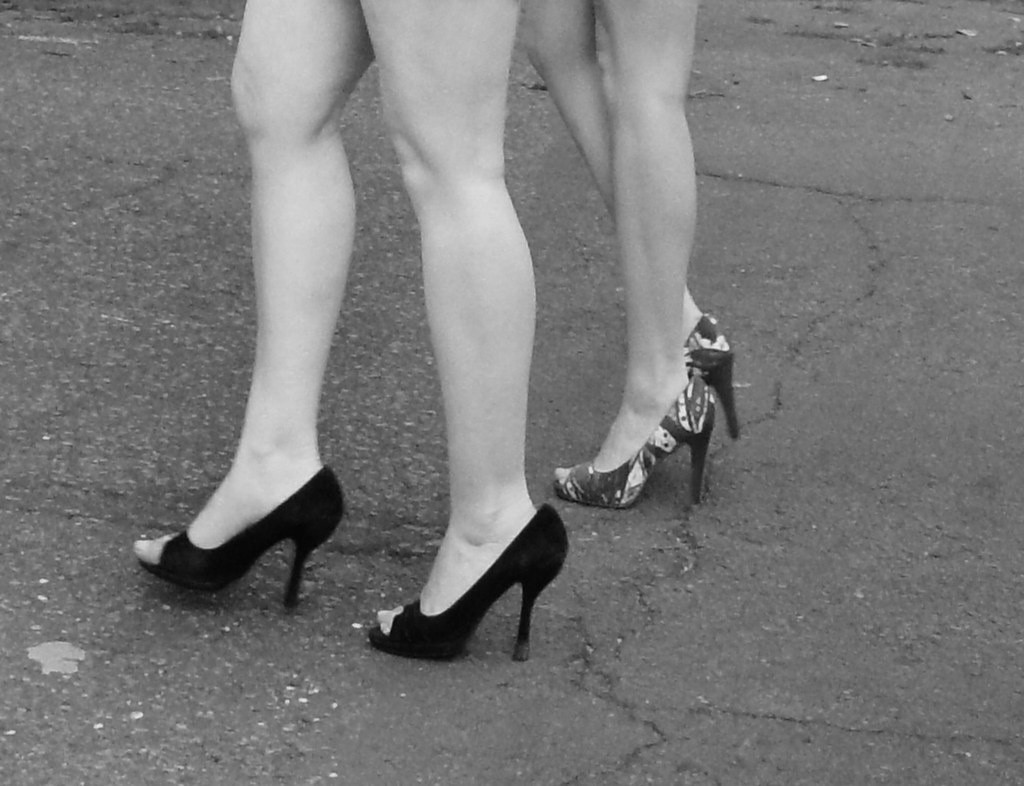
[681,316,738,438]
[551,377,716,508]
[368,504,569,659]
[136,467,341,608]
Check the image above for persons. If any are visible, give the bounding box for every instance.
[516,0,742,509]
[133,0,571,667]
